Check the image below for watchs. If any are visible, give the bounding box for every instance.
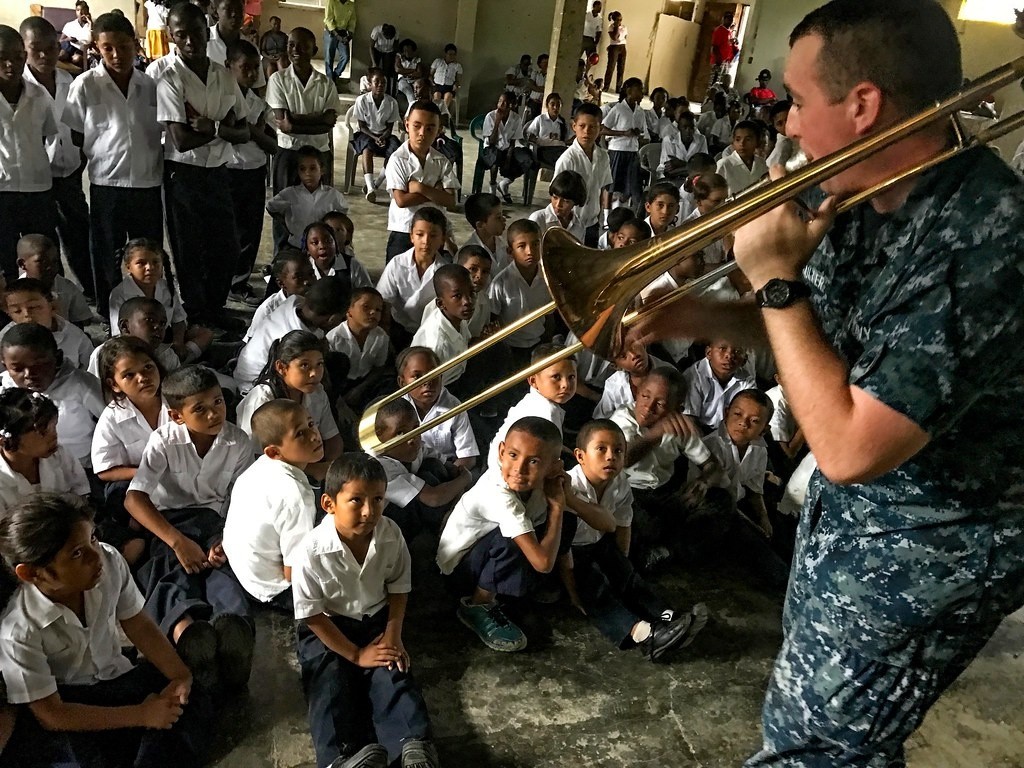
[754,278,810,309]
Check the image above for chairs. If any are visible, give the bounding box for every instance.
[470,113,556,207]
[638,143,662,187]
[344,103,463,204]
[390,75,461,130]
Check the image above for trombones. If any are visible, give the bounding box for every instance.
[356,51,1022,463]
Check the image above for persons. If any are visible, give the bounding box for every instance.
[0,0,829,768]
[628,0,1024,768]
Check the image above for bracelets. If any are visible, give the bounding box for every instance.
[214,120,221,136]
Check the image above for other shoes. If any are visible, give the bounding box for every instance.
[365,191,377,203]
[361,179,380,195]
[175,621,220,692]
[401,740,438,768]
[497,184,513,204]
[212,612,252,689]
[186,321,239,342]
[226,283,264,307]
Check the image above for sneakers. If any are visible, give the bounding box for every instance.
[636,614,691,662]
[330,744,388,768]
[455,597,528,652]
[675,602,708,651]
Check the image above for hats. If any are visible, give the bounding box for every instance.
[755,69,771,81]
